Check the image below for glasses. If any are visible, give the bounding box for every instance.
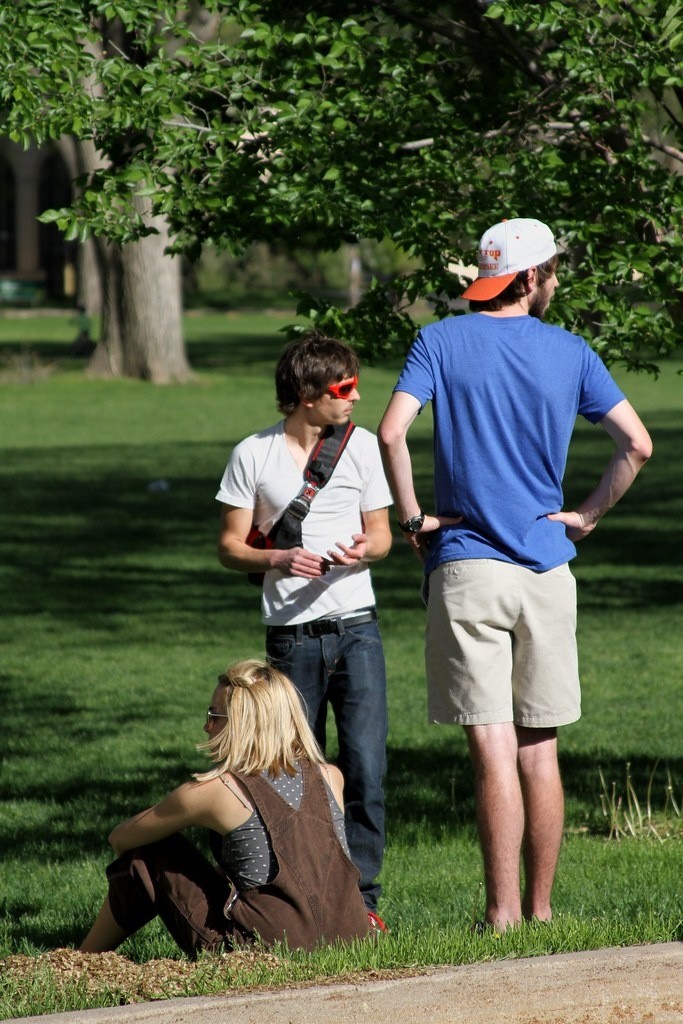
[203,711,227,727]
[322,374,360,399]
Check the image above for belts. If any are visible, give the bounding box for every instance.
[264,611,375,637]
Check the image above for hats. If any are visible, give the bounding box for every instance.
[459,216,557,300]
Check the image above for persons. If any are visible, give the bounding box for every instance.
[216,335,394,940]
[79,659,371,964]
[377,215,655,937]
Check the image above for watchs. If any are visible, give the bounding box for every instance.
[396,509,425,534]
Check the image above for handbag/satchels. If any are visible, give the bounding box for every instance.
[243,523,269,585]
